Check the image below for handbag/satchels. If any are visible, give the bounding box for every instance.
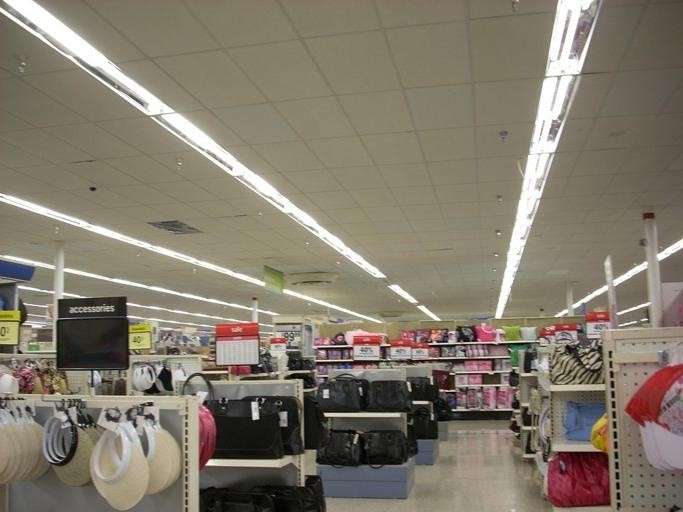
[365,381,413,412]
[549,341,605,385]
[285,374,316,389]
[243,395,304,455]
[200,487,276,512]
[252,347,277,373]
[316,374,363,413]
[413,384,438,402]
[278,485,322,511]
[301,359,313,369]
[288,358,300,370]
[406,377,430,384]
[407,407,438,439]
[525,336,550,373]
[364,430,408,469]
[305,474,327,512]
[547,452,610,507]
[304,396,329,450]
[316,430,362,466]
[564,401,606,441]
[202,400,284,459]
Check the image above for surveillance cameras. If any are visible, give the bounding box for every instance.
[88,185,97,192]
[639,238,646,246]
[252,297,257,300]
[540,308,544,311]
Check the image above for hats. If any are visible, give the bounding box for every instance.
[0,364,20,394]
[173,367,188,381]
[92,423,149,510]
[42,407,105,487]
[123,415,181,495]
[133,365,155,392]
[156,366,174,393]
[624,365,682,471]
[0,405,51,484]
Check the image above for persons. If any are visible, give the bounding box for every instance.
[332,333,348,345]
